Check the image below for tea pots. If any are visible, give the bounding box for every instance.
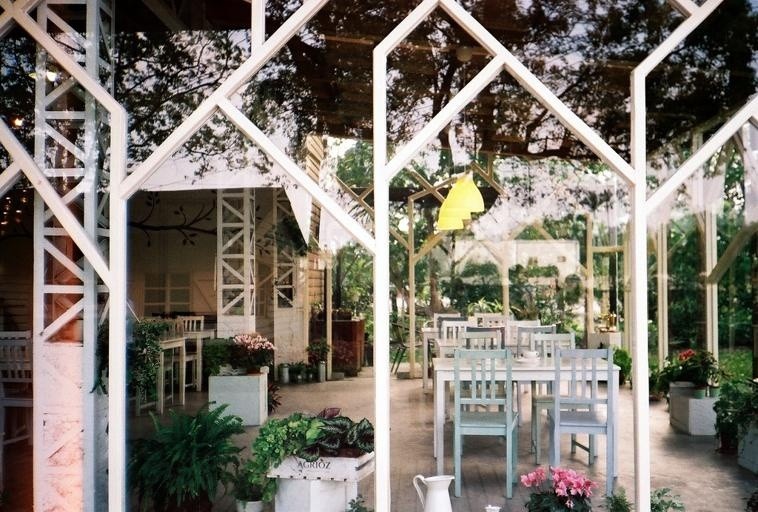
[412,475,455,512]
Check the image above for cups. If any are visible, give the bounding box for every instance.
[484,504,501,512]
[522,351,540,358]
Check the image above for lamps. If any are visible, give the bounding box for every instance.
[437,175,486,230]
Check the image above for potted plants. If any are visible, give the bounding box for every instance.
[309,304,359,321]
[90,312,172,398]
[642,351,758,456]
[289,338,352,382]
[252,406,374,483]
[229,463,266,512]
[613,347,631,385]
[126,401,247,512]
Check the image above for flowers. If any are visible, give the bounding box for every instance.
[520,464,598,510]
[203,329,275,376]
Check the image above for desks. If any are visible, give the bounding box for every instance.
[431,357,622,478]
[419,327,556,419]
[154,329,219,414]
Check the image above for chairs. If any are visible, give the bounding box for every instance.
[176,315,204,335]
[530,333,596,465]
[434,312,556,350]
[548,347,615,498]
[0,331,32,454]
[457,331,501,350]
[391,323,432,376]
[454,349,520,498]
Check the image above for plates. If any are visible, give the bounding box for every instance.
[515,359,542,364]
